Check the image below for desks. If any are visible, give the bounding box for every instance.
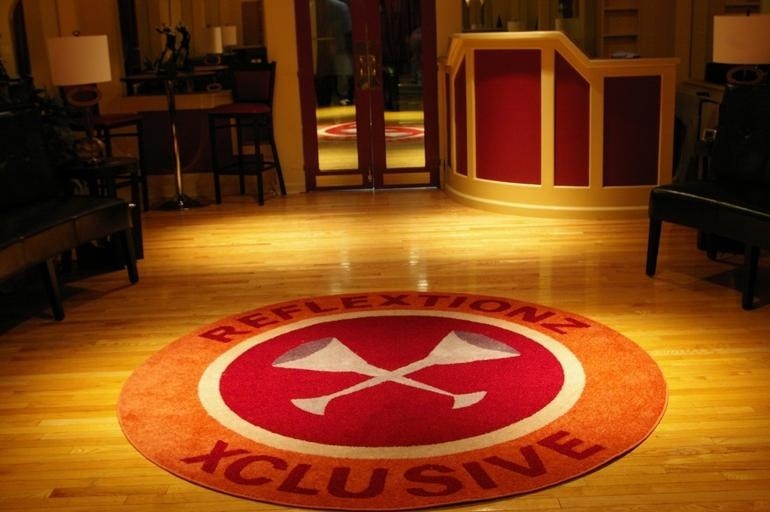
[119,63,230,211]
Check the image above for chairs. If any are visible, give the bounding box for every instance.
[1,104,140,323]
[203,60,289,205]
[646,81,770,311]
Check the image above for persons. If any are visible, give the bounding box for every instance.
[173,21,192,61]
[314,0,353,110]
[155,22,178,63]
[379,0,412,111]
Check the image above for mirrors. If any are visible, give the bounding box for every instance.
[117,0,264,76]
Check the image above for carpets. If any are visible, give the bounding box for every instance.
[117,288,668,510]
[314,120,426,143]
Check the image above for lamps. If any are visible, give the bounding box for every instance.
[44,29,113,165]
[711,13,770,82]
